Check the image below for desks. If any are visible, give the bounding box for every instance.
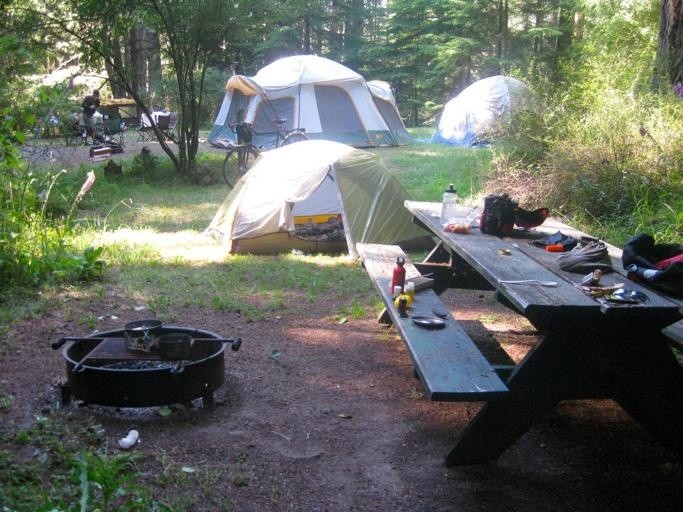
[405,200,682,466]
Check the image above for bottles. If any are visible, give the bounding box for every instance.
[406,281,415,301]
[259,144,267,152]
[439,184,458,227]
[391,256,406,300]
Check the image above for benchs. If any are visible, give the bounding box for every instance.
[356,242,510,403]
[662,320,682,352]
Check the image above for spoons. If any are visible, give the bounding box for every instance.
[498,279,559,287]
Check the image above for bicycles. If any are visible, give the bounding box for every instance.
[222,118,311,190]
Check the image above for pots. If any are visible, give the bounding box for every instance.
[158,333,191,360]
[124,319,163,349]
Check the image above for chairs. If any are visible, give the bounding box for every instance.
[63,104,181,146]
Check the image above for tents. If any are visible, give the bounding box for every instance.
[430,75,545,149]
[201,139,432,262]
[206,54,410,151]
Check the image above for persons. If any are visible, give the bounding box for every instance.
[80,90,100,146]
[234,109,254,172]
